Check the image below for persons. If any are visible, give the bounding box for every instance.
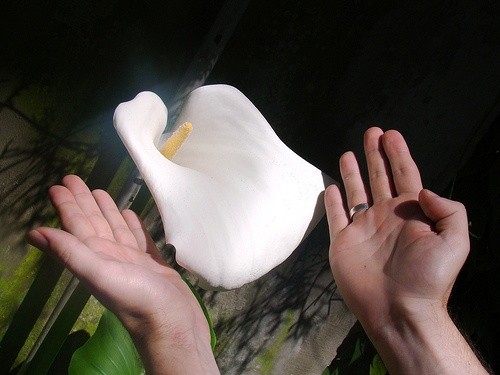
[23,124,493,374]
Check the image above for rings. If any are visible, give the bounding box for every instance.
[347,204,371,218]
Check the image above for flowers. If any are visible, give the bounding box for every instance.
[112,82,342,293]
